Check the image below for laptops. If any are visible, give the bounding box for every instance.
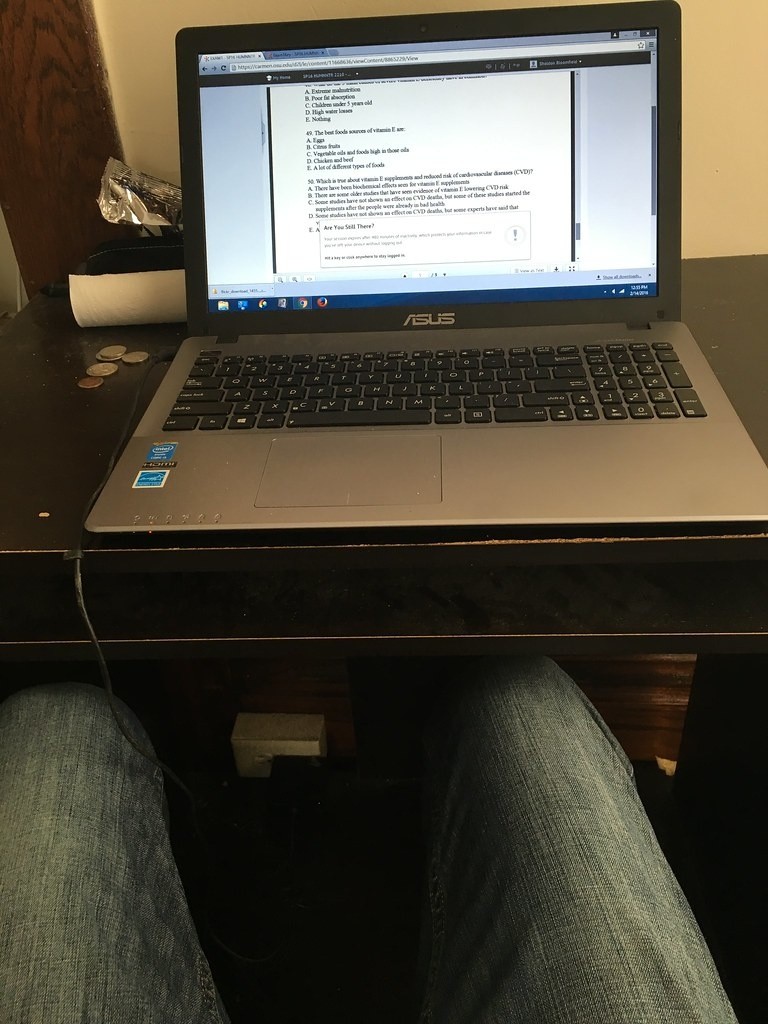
[85,1,768,532]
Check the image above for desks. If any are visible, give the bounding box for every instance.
[0,254,768,993]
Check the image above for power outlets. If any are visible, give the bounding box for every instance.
[230,711,327,787]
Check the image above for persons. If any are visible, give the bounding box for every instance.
[1,652,739,1023]
